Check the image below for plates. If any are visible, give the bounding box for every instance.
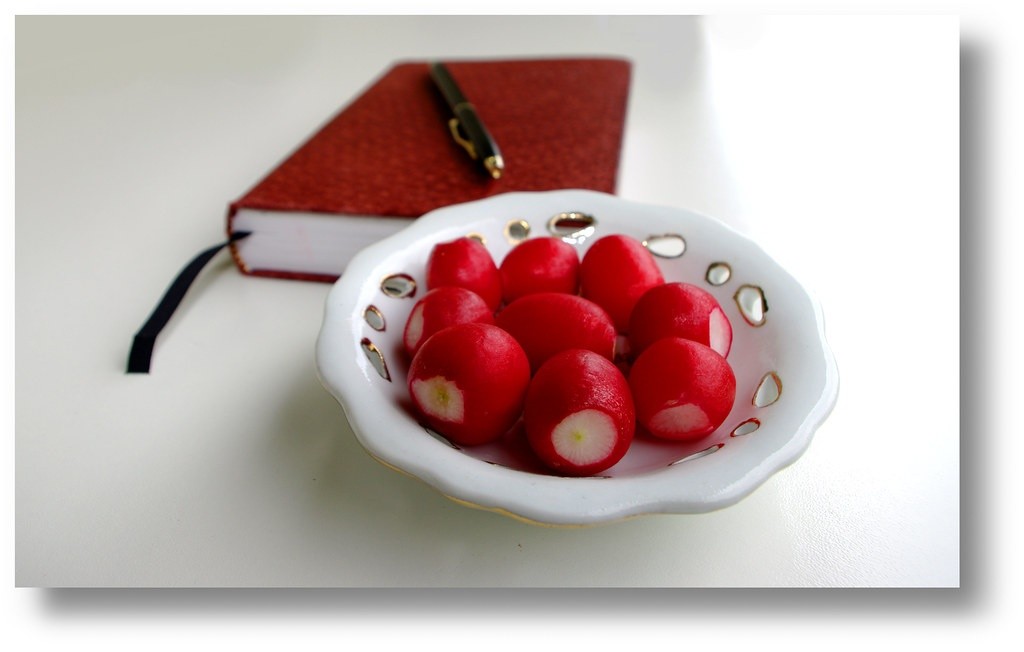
[314,188,841,529]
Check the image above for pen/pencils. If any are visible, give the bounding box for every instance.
[423,60,505,178]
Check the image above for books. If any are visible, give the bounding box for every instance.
[226,57,635,284]
